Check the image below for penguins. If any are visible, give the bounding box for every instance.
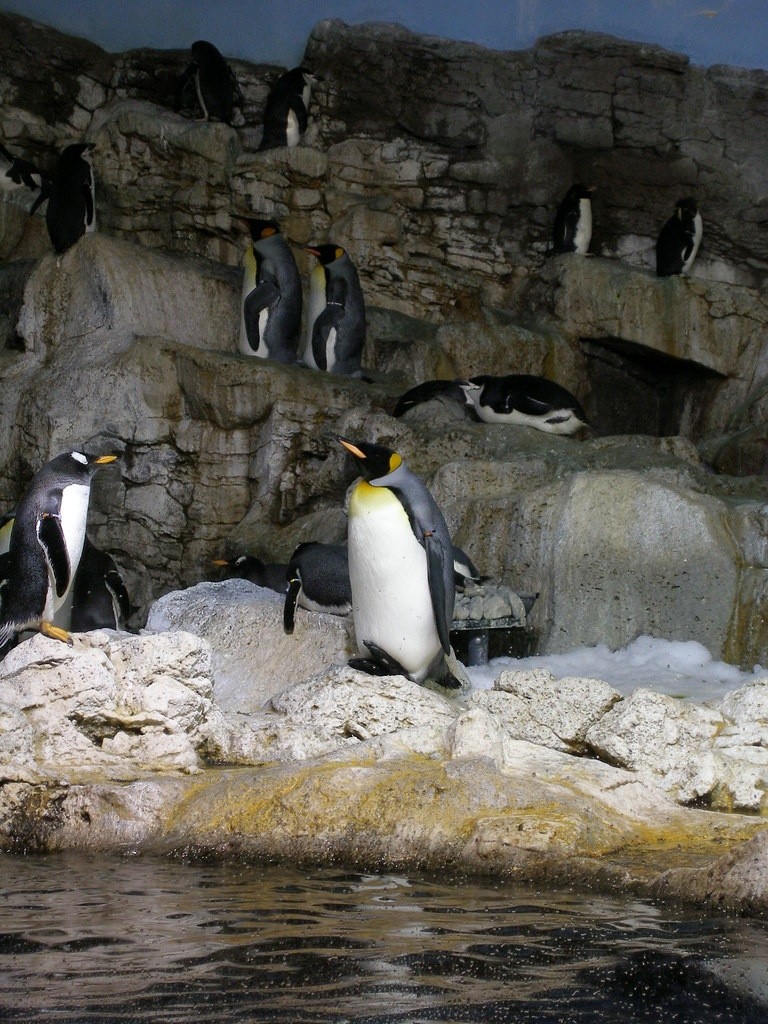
[229,213,303,363]
[339,435,480,698]
[189,40,236,123]
[546,183,598,257]
[254,66,326,154]
[655,196,703,277]
[212,555,268,588]
[282,539,353,632]
[28,141,97,255]
[0,449,142,648]
[461,375,590,436]
[304,243,369,378]
